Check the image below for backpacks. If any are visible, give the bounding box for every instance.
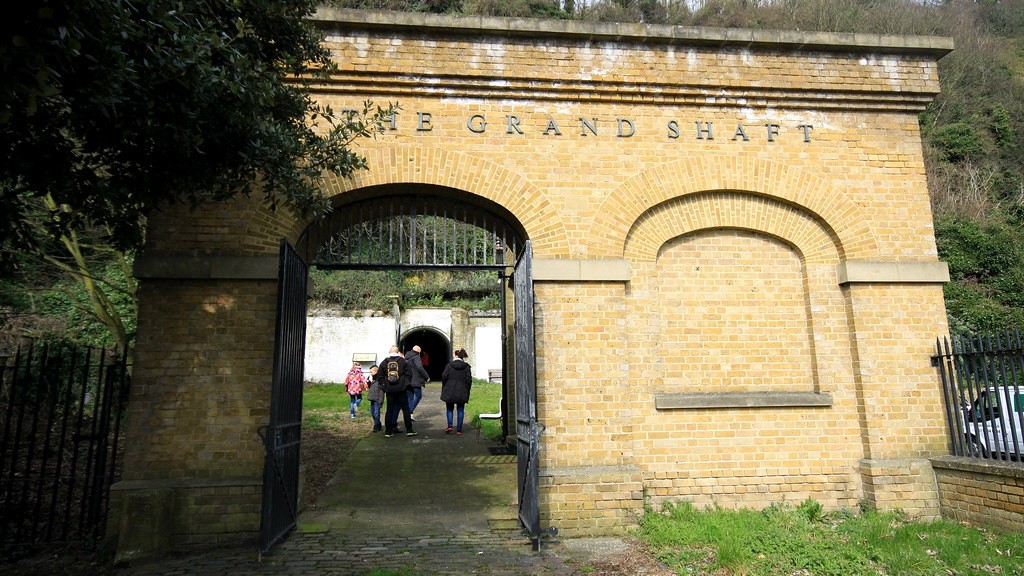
[349,372,362,396]
[386,357,401,383]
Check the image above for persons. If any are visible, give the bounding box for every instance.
[390,345,404,434]
[366,365,384,434]
[440,348,472,435]
[344,362,367,419]
[377,346,418,438]
[405,344,430,421]
[421,350,430,381]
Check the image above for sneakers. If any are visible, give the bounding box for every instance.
[457,429,461,434]
[354,403,358,413]
[373,414,418,438]
[351,414,357,418]
[445,426,453,432]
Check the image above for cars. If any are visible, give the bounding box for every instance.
[957,385,1024,459]
